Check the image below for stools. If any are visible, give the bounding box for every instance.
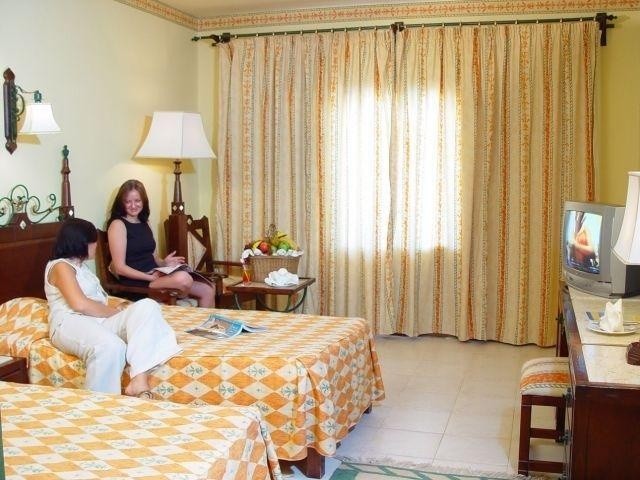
[518,357,571,477]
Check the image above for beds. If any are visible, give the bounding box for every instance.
[0,144,385,479]
[0,380,281,479]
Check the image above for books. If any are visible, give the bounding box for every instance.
[184,313,267,341]
[146,263,217,289]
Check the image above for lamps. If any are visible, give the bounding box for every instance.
[134,111,217,266]
[609,171,640,367]
[3,67,64,155]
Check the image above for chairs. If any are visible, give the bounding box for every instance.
[164,215,256,309]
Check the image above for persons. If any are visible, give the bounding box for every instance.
[106,179,217,307]
[43,218,180,400]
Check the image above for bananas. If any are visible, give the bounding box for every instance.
[253,240,262,250]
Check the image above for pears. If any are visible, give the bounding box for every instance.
[278,242,288,251]
[271,245,277,252]
[284,240,291,249]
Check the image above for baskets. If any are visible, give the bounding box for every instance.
[246,255,302,283]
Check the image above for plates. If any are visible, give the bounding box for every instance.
[586,321,639,335]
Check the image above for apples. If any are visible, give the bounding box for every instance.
[259,241,270,253]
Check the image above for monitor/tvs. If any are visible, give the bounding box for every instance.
[559,202,640,301]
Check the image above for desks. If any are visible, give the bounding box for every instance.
[225,276,316,312]
[556,277,640,479]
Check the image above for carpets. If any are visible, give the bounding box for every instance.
[328,456,568,480]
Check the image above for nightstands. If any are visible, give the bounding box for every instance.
[0,357,29,384]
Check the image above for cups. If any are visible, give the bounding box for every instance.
[241,265,256,285]
